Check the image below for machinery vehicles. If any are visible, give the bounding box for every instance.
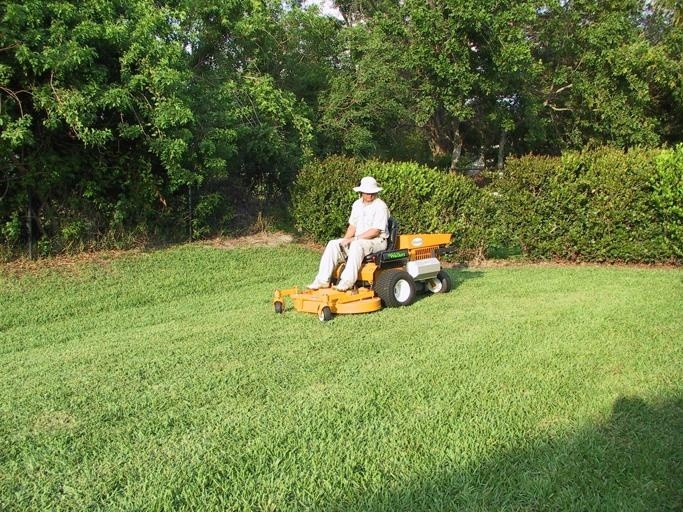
[271,216,453,321]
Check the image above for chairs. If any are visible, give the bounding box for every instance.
[345,218,397,263]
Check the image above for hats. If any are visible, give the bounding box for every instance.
[352,176,384,194]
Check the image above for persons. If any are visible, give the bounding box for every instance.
[302,175,392,293]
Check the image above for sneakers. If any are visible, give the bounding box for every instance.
[335,280,354,291]
[307,279,330,289]
